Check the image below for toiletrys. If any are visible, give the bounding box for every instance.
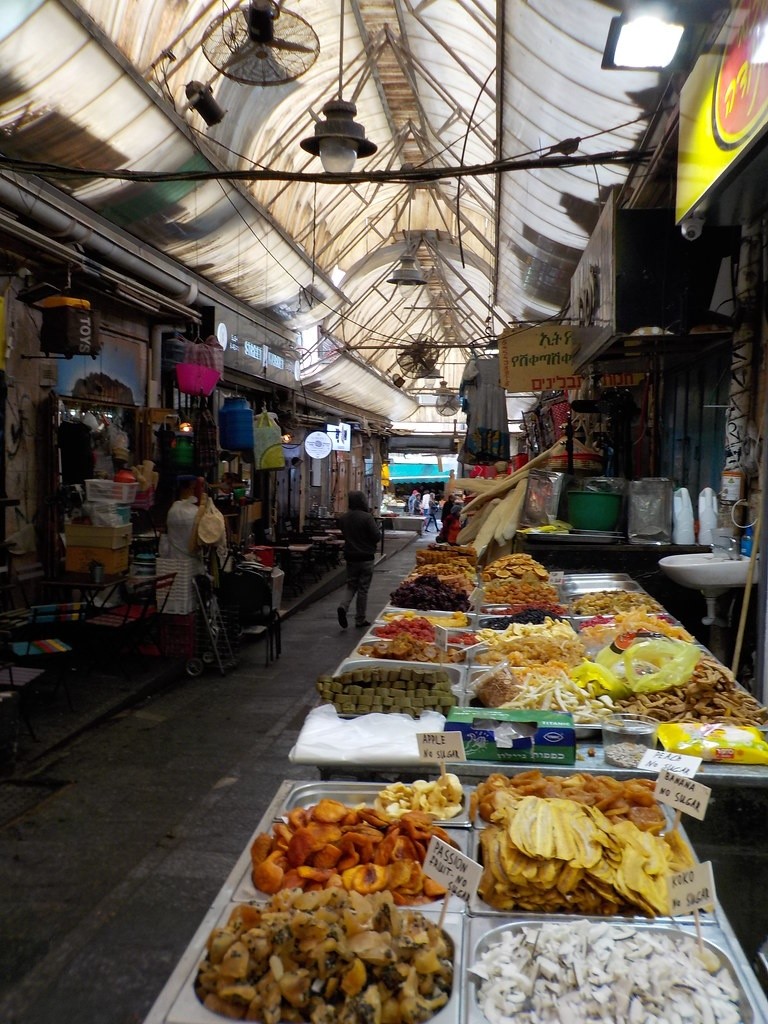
[740,523,754,557]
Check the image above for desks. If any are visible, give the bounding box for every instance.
[270,528,346,597]
[37,572,130,610]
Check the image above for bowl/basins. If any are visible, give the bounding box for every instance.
[560,489,625,531]
[176,364,220,396]
[601,713,661,770]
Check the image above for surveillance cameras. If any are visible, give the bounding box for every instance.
[681,217,703,240]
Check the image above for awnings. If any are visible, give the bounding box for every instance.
[388,463,450,485]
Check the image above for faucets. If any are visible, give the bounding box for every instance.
[710,535,740,560]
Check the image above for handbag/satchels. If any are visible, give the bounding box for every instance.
[357,550,375,577]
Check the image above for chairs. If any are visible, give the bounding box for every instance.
[0,571,177,743]
[234,563,282,668]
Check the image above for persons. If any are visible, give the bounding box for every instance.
[408,490,419,515]
[336,490,382,628]
[160,474,210,678]
[440,488,467,547]
[208,472,249,494]
[413,493,422,515]
[421,490,430,526]
[424,493,440,532]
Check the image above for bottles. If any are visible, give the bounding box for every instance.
[740,524,753,557]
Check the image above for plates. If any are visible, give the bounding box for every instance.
[538,528,625,545]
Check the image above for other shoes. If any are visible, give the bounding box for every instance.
[437,530,440,532]
[337,607,348,628]
[355,620,371,628]
[425,529,429,532]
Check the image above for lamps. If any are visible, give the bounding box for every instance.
[300,1,378,175]
[385,162,426,298]
[601,0,689,72]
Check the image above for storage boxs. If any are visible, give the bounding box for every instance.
[83,477,139,503]
[67,546,130,575]
[65,520,133,550]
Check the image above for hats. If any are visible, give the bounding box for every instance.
[451,506,462,514]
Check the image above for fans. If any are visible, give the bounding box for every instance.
[436,394,460,416]
[395,332,440,380]
[200,0,320,88]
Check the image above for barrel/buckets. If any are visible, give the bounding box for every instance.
[711,527,734,558]
[673,486,718,545]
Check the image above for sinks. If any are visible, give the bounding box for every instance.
[658,553,758,588]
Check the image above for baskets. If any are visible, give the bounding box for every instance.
[85,480,139,504]
[154,557,199,615]
